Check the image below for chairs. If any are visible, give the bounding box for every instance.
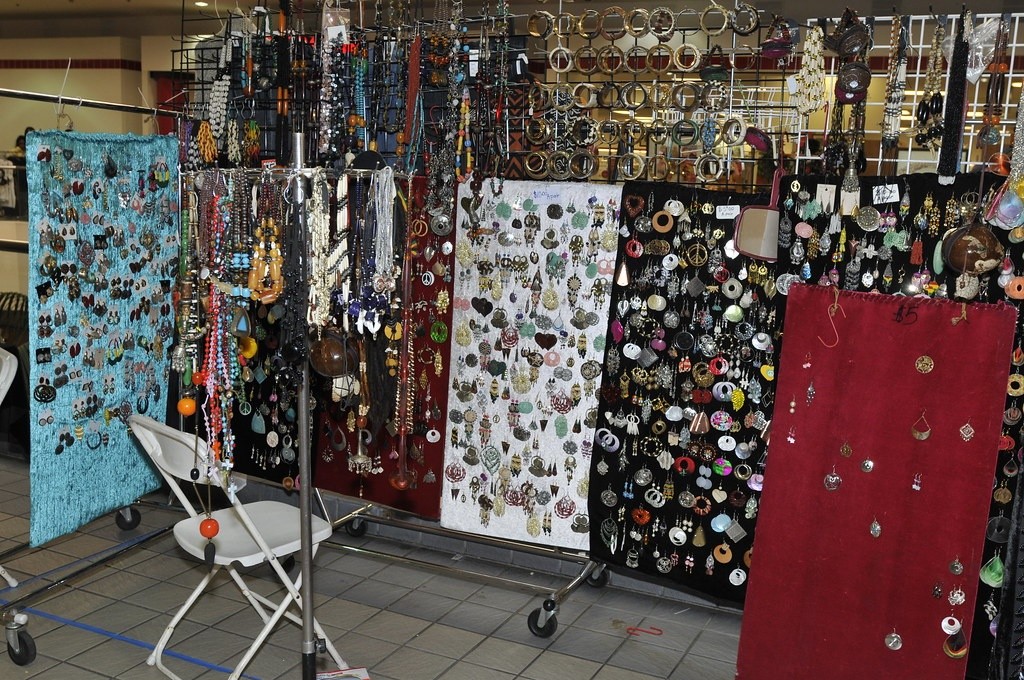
[125,412,348,680]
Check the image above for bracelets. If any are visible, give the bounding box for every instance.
[524,3,761,183]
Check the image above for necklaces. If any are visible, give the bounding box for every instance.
[171,0,510,573]
[795,8,1012,193]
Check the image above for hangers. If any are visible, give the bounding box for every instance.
[508,52,528,82]
